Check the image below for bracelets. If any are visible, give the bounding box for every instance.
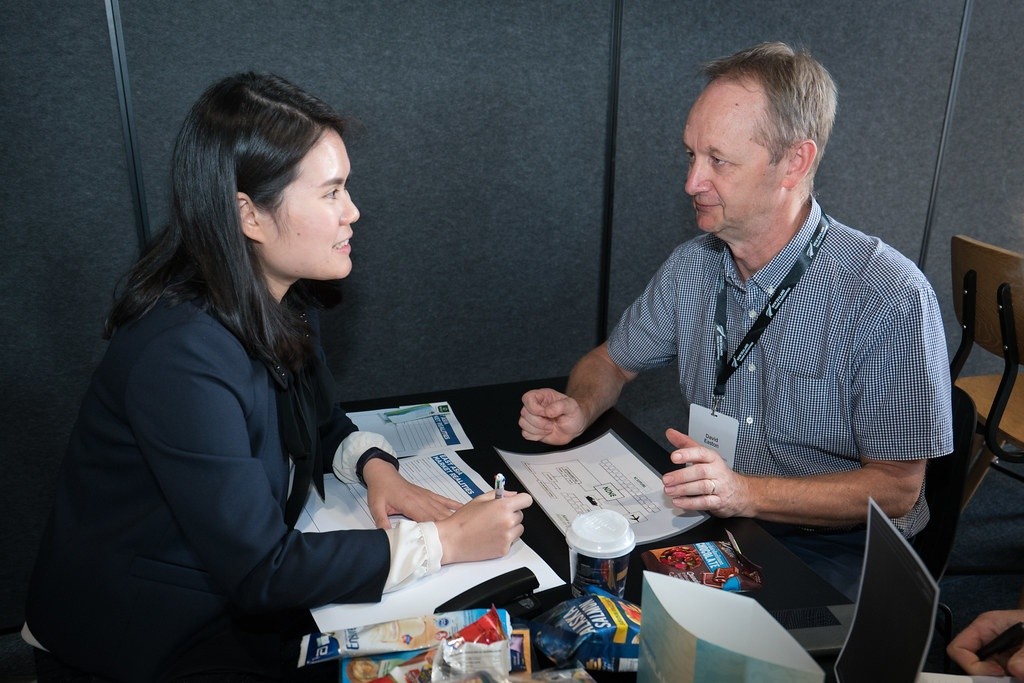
[356,447,400,489]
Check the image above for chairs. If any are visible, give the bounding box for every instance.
[942,235,1023,517]
[902,382,976,647]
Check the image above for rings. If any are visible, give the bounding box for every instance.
[711,480,716,494]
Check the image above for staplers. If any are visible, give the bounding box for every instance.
[433,566,546,624]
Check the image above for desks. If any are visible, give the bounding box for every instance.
[325,375,963,683]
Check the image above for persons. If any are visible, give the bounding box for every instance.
[947,609,1024,683]
[519,42,954,595]
[19,72,534,683]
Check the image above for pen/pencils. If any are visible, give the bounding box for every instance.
[975,621,1024,662]
[494,474,506,501]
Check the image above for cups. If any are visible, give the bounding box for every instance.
[566,509,636,601]
